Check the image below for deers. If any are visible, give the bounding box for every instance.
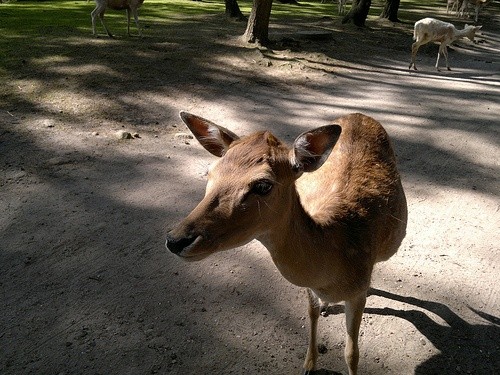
[408,18,483,73]
[87,0,145,39]
[446,0,491,22]
[163,110,407,375]
